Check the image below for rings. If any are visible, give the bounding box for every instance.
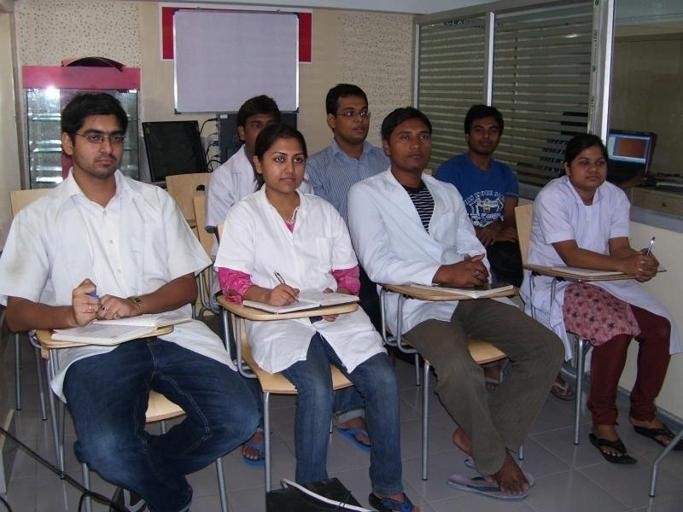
[88,303,92,312]
[101,305,108,313]
[473,270,481,277]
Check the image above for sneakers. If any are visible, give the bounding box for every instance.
[109,485,147,512]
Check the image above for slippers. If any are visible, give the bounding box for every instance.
[447,474,528,499]
[464,455,535,487]
[634,424,683,450]
[338,423,371,450]
[242,435,272,466]
[588,431,637,464]
[368,492,414,512]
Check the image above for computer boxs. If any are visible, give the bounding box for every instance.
[217,111,297,164]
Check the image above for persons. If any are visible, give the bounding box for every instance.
[213,123,419,511]
[520,133,683,465]
[204,96,315,314]
[433,103,525,289]
[305,83,390,229]
[348,106,565,494]
[0,93,260,512]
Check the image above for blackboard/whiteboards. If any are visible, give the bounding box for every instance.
[173,10,300,115]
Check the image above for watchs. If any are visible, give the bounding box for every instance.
[127,295,144,315]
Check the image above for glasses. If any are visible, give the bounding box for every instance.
[65,130,126,143]
[333,111,371,118]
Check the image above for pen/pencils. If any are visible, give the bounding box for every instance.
[646,237,655,256]
[273,270,301,304]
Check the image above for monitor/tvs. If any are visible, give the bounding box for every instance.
[141,120,209,182]
[607,128,657,175]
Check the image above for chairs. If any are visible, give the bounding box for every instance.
[378,283,524,480]
[9,189,227,512]
[515,204,667,446]
[195,189,221,322]
[166,174,218,317]
[206,225,359,491]
